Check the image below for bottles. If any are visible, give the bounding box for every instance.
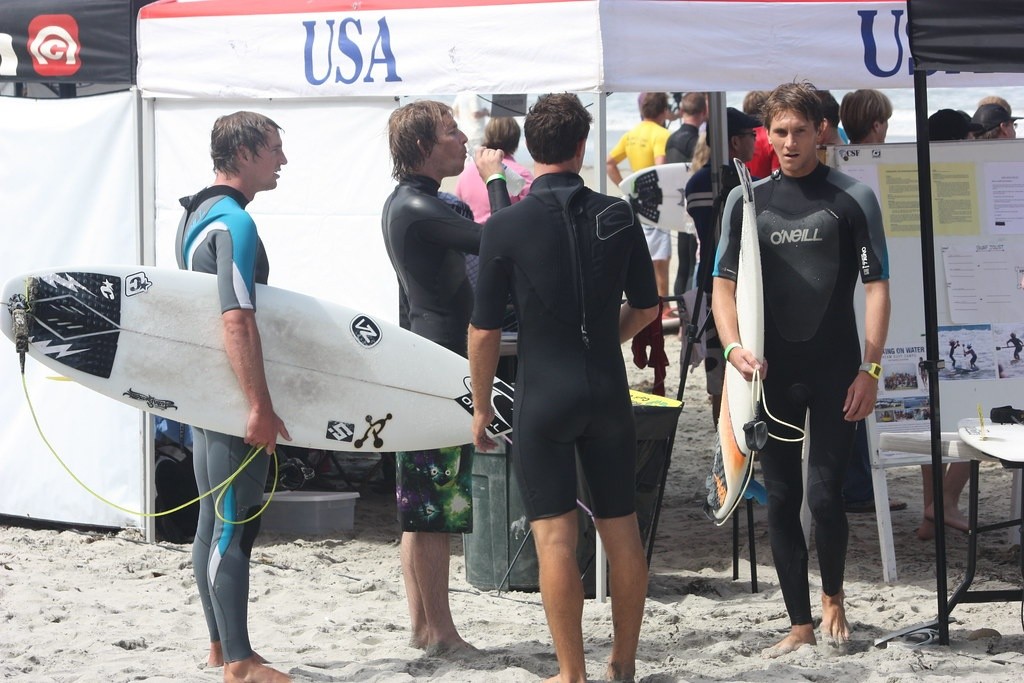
[466,145,526,196]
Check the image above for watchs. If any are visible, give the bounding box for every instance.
[859,362,882,380]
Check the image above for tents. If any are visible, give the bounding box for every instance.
[0,0,914,547]
[910,0,1024,547]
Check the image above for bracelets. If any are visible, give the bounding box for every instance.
[486,174,508,190]
[724,343,743,362]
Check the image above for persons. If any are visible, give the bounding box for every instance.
[175,112,312,683]
[467,89,660,683]
[381,99,512,661]
[712,74,892,659]
[456,116,536,224]
[606,84,1024,540]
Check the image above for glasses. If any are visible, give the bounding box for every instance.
[735,130,756,139]
[1002,122,1018,130]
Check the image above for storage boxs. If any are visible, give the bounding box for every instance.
[260,489,360,536]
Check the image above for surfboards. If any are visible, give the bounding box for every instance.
[617,160,695,236]
[702,158,770,528]
[0,261,515,453]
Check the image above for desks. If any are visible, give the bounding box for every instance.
[879,431,1024,629]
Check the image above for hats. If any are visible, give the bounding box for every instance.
[929,109,983,139]
[707,107,763,129]
[972,104,1023,134]
